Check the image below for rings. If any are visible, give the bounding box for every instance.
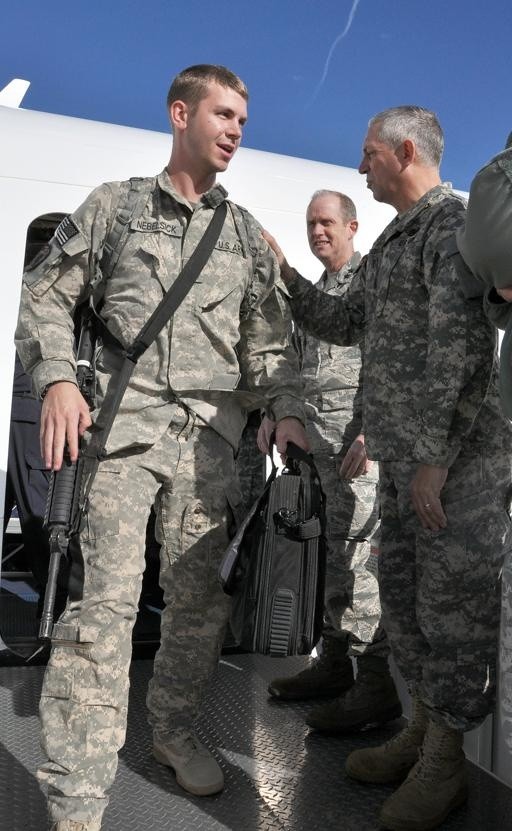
[422,502,433,511]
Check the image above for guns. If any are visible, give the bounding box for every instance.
[39,297,93,641]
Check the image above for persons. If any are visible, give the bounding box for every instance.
[12,63,319,831]
[5,347,70,628]
[259,188,411,734]
[450,118,511,425]
[260,103,499,830]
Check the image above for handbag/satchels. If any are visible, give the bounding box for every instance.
[220,442,328,658]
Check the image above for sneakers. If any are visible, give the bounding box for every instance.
[154,729,225,795]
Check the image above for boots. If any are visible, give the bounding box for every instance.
[268,640,355,702]
[305,658,403,731]
[344,699,429,786]
[380,719,470,828]
